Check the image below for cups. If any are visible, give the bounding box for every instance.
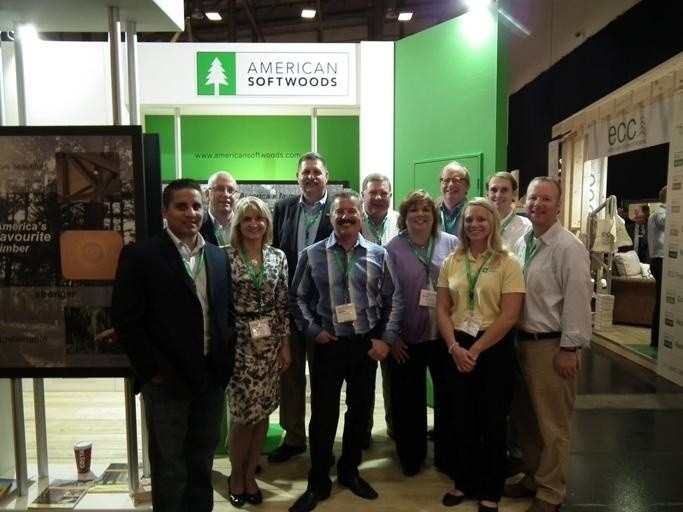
[74,443,93,474]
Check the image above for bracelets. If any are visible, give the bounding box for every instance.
[447,341,461,356]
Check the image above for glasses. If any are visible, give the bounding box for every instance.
[209,186,238,195]
[440,177,467,184]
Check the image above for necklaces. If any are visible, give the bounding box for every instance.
[246,249,262,269]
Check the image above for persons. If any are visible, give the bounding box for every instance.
[615,207,633,252]
[269,154,362,465]
[435,197,526,512]
[200,171,243,451]
[384,190,465,476]
[630,212,649,262]
[647,185,667,348]
[641,205,650,220]
[216,196,292,507]
[510,178,591,510]
[293,190,404,512]
[425,162,475,442]
[486,172,533,268]
[110,178,236,512]
[350,176,404,451]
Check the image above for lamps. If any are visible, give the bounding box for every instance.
[396,0,414,23]
[300,0,316,19]
[204,0,223,21]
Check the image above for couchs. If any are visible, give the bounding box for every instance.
[612,251,656,326]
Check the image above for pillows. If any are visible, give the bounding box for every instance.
[615,251,641,276]
[620,274,641,278]
[640,262,650,278]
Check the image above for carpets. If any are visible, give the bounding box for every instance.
[214,424,284,458]
[625,344,657,360]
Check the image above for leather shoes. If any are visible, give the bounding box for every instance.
[245,487,263,504]
[526,498,560,511]
[339,472,379,500]
[442,488,467,505]
[287,486,330,512]
[477,497,498,511]
[228,476,246,507]
[269,443,306,462]
[503,483,532,496]
